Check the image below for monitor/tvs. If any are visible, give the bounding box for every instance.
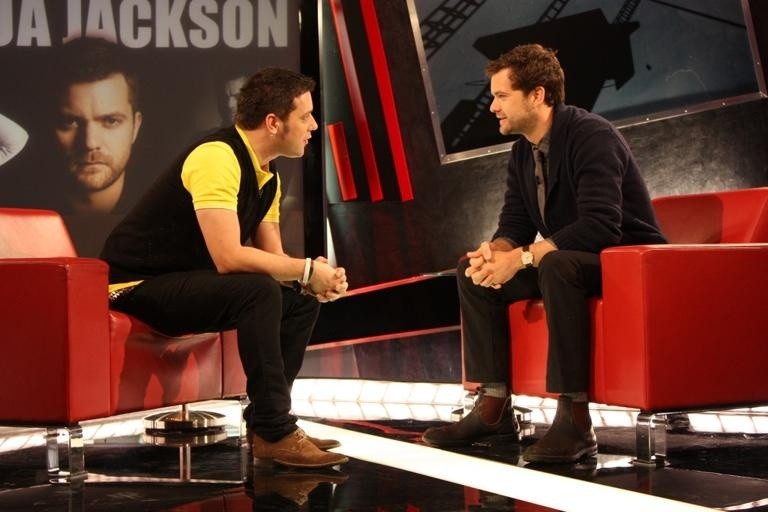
[0,0,329,269]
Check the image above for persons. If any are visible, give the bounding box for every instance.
[0,34,156,259]
[99,67,350,469]
[421,42,668,464]
[183,67,294,200]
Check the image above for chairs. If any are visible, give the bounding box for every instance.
[0,206,252,499]
[457,186,768,467]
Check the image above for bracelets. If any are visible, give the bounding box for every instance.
[302,257,313,287]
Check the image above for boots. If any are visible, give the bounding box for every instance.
[522,390,599,462]
[422,386,521,446]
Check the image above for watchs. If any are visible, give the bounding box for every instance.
[520,244,534,269]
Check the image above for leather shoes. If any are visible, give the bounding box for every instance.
[252,470,348,504]
[246,429,349,468]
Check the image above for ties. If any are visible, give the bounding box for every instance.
[533,146,549,232]
